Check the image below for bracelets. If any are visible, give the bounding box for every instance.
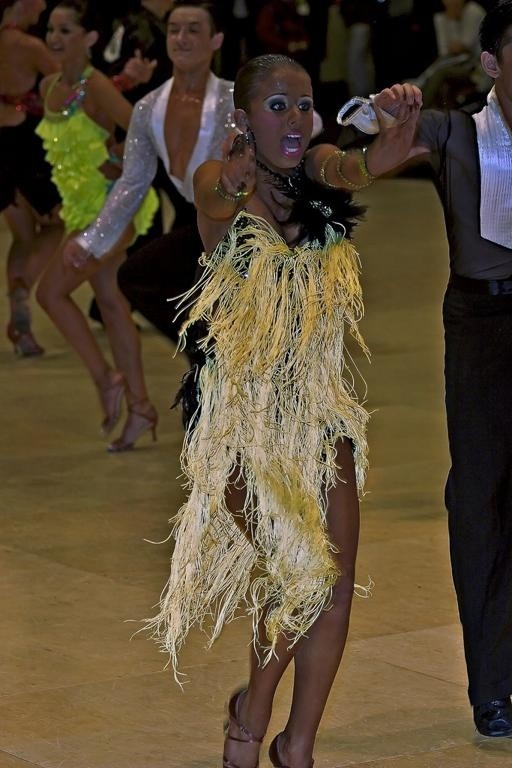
[316,144,377,194]
[206,170,253,210]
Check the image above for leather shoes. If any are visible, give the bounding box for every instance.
[473,695,512,737]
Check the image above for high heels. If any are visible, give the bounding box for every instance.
[7,321,44,357]
[222,689,266,768]
[106,395,157,452]
[94,366,132,441]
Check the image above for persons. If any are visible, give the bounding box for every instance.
[193,51,432,765]
[335,1,508,741]
[0,1,512,457]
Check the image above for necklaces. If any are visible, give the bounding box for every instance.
[252,158,307,190]
